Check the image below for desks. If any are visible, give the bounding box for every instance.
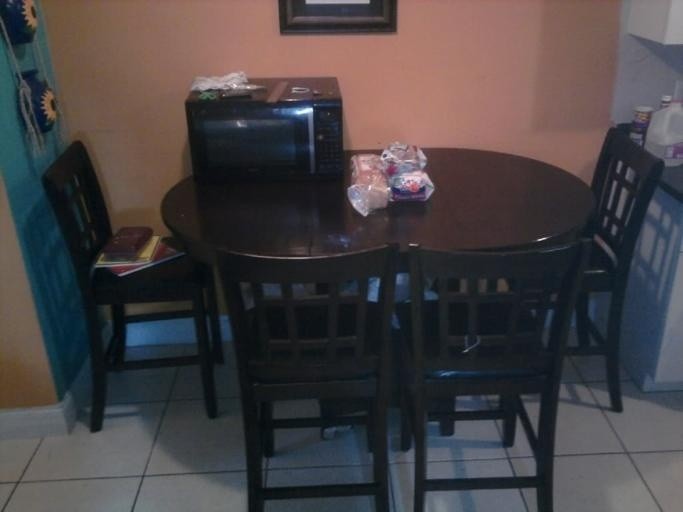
[160,147,596,457]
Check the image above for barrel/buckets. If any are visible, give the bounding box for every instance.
[643,99,683,168]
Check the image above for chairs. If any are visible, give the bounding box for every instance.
[401,243,579,511]
[565,129,665,409]
[219,244,397,512]
[43,140,222,433]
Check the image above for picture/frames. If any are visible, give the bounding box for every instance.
[277,0,397,36]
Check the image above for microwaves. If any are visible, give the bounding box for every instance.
[185,76,345,187]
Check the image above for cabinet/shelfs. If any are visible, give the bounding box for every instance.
[586,147,682,393]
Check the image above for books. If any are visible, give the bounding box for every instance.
[93,226,186,282]
